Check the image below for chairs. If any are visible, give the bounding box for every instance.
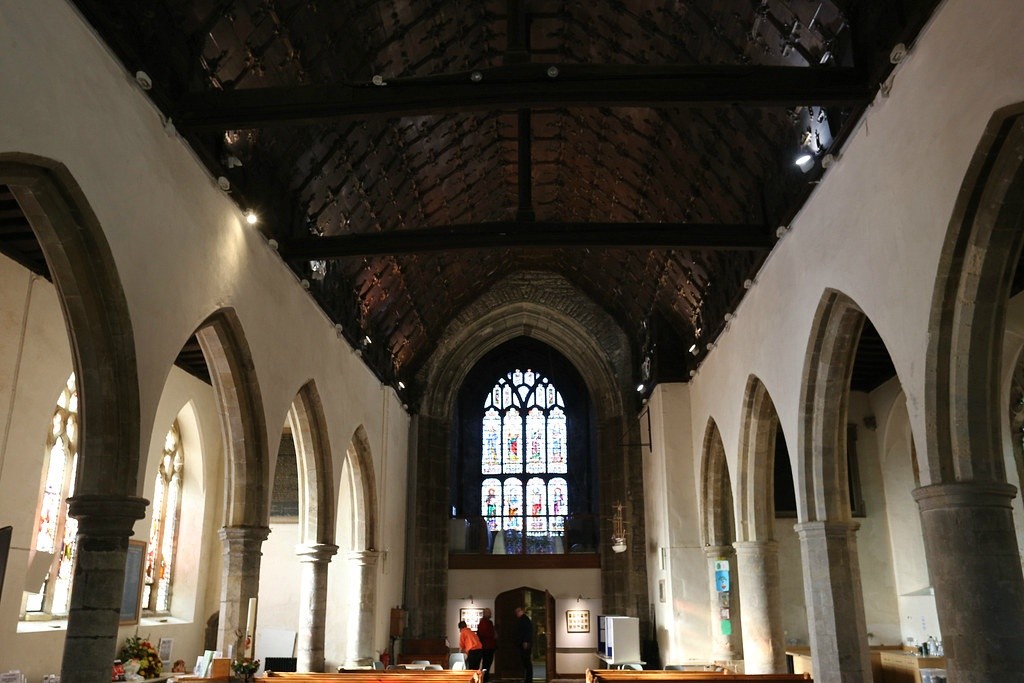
[372,652,467,671]
[622,664,643,671]
[664,664,683,670]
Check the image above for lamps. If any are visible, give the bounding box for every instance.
[547,43,910,407]
[131,62,483,391]
[612,504,627,554]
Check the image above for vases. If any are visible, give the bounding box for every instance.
[235,673,254,683]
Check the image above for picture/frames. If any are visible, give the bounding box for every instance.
[158,638,174,662]
[459,607,485,632]
[567,609,590,632]
[119,540,147,625]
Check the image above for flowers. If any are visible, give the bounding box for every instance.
[231,658,260,673]
[120,626,163,675]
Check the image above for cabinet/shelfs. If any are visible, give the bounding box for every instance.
[784,644,946,683]
[594,614,647,670]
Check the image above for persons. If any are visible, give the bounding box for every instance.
[511,606,533,683]
[477,608,496,683]
[458,621,483,670]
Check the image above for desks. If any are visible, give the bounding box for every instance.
[398,663,440,669]
[112,672,185,683]
